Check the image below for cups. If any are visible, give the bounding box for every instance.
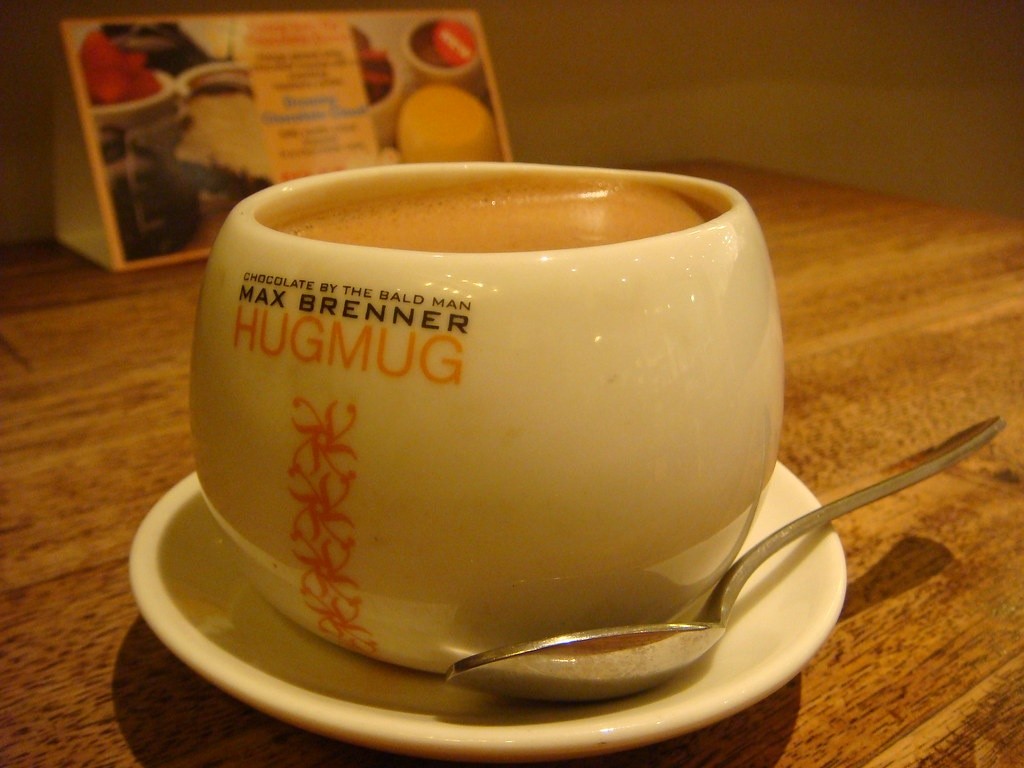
[189,161,785,674]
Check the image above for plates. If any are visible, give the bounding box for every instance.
[128,460,847,763]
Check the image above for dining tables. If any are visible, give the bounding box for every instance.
[0,158,1024,768]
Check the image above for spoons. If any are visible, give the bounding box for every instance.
[442,415,1006,700]
